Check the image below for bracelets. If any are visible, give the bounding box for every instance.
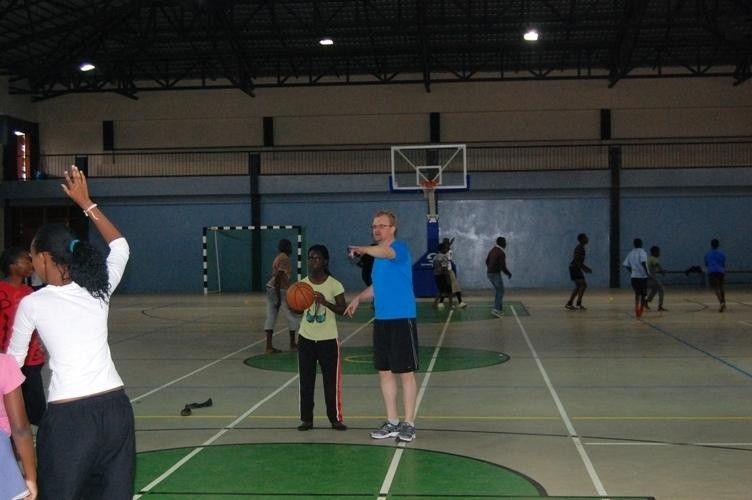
[82,203,98,218]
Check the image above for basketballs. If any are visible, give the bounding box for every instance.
[287,282,315,310]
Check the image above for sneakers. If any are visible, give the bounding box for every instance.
[368,419,400,439]
[576,305,584,309]
[332,421,347,430]
[395,422,416,442]
[490,308,504,319]
[297,422,313,431]
[565,304,575,310]
[438,303,444,307]
[457,302,466,308]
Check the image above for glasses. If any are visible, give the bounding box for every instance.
[370,224,391,229]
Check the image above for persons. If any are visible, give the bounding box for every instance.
[644,245,667,312]
[354,243,381,310]
[703,238,729,312]
[284,244,347,431]
[430,237,468,310]
[342,208,419,443]
[564,232,593,311]
[262,238,302,353]
[622,237,651,316]
[485,237,512,318]
[5,162,137,500]
[0,246,53,500]
